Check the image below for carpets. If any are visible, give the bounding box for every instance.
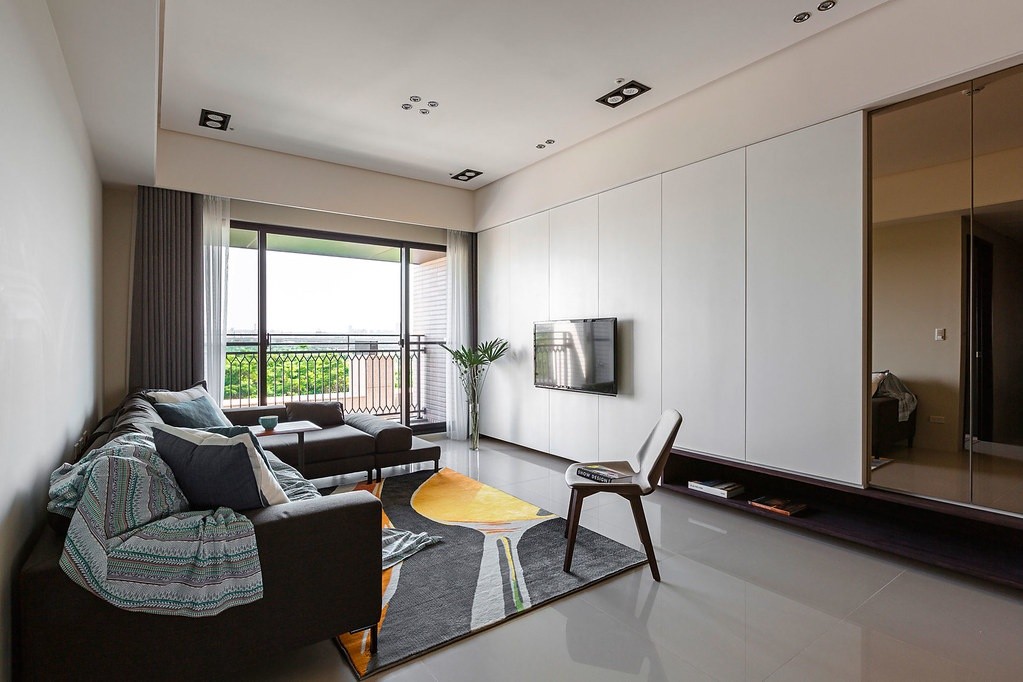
[331,467,649,682]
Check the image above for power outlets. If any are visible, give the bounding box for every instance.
[929,416,944,423]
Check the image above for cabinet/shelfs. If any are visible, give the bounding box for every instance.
[661,450,1023,590]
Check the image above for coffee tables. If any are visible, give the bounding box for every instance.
[248,420,322,476]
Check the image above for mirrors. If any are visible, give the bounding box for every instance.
[867,63,1023,518]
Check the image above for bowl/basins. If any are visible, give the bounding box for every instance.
[261,416,279,430]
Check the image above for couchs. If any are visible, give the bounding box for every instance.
[872,373,917,459]
[12,400,442,682]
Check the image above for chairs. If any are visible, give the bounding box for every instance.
[562,408,683,582]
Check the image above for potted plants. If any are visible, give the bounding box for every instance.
[438,337,509,450]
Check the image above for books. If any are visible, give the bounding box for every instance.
[577,465,633,484]
[748,496,806,515]
[688,481,744,499]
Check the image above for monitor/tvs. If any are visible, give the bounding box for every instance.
[534,317,618,397]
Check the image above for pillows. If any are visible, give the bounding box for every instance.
[149,424,291,512]
[147,387,233,427]
[284,402,345,426]
[872,369,889,398]
[154,396,229,427]
